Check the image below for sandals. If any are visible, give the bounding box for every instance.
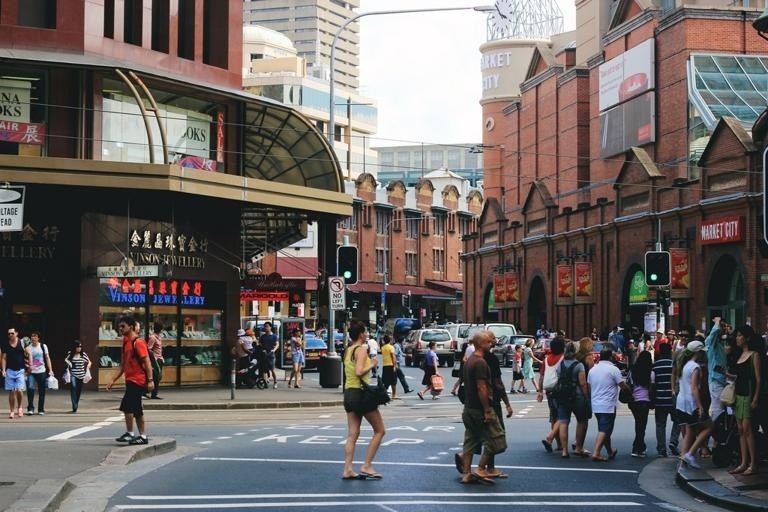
[431,396,442,400]
[417,391,425,400]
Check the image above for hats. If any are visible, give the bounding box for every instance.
[685,341,708,352]
[292,327,301,334]
[236,328,246,336]
[656,329,663,334]
[246,328,254,336]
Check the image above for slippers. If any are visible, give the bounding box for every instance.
[607,448,618,459]
[574,449,592,456]
[591,455,609,463]
[454,453,464,473]
[488,470,509,479]
[561,452,569,457]
[342,473,359,481]
[358,471,383,481]
[541,438,553,452]
[470,471,497,485]
[459,474,479,484]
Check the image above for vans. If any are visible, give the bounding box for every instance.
[381,318,420,347]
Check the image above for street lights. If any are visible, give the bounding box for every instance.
[329,3,503,151]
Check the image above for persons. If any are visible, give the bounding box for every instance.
[367,335,475,400]
[341,319,384,479]
[514,317,767,477]
[65,338,91,412]
[26,332,54,415]
[236,322,306,390]
[454,330,513,478]
[0,327,32,419]
[106,317,154,445]
[146,321,166,400]
[460,330,507,484]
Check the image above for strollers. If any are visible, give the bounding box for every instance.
[232,351,268,389]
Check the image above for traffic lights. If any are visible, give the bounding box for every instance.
[643,250,670,286]
[339,249,358,284]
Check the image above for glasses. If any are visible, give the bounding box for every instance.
[7,332,15,335]
[75,345,82,347]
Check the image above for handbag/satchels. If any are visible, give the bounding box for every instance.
[419,358,427,369]
[61,368,72,384]
[719,383,737,406]
[360,382,392,407]
[430,374,444,391]
[451,369,460,377]
[618,382,634,405]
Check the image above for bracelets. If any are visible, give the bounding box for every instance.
[148,379,153,382]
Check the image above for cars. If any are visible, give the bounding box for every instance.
[284,335,328,370]
[407,328,456,367]
[447,324,480,357]
[533,337,571,367]
[496,333,535,368]
[591,342,624,366]
[476,324,518,357]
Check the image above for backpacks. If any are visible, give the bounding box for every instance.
[552,360,578,407]
[542,356,559,390]
[131,337,163,388]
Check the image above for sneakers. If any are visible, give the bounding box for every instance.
[656,449,667,458]
[17,408,24,417]
[115,432,135,443]
[668,443,680,455]
[680,453,701,470]
[8,411,15,419]
[128,434,149,445]
[728,464,746,473]
[38,410,44,415]
[27,409,34,415]
[741,465,760,476]
[630,446,647,457]
